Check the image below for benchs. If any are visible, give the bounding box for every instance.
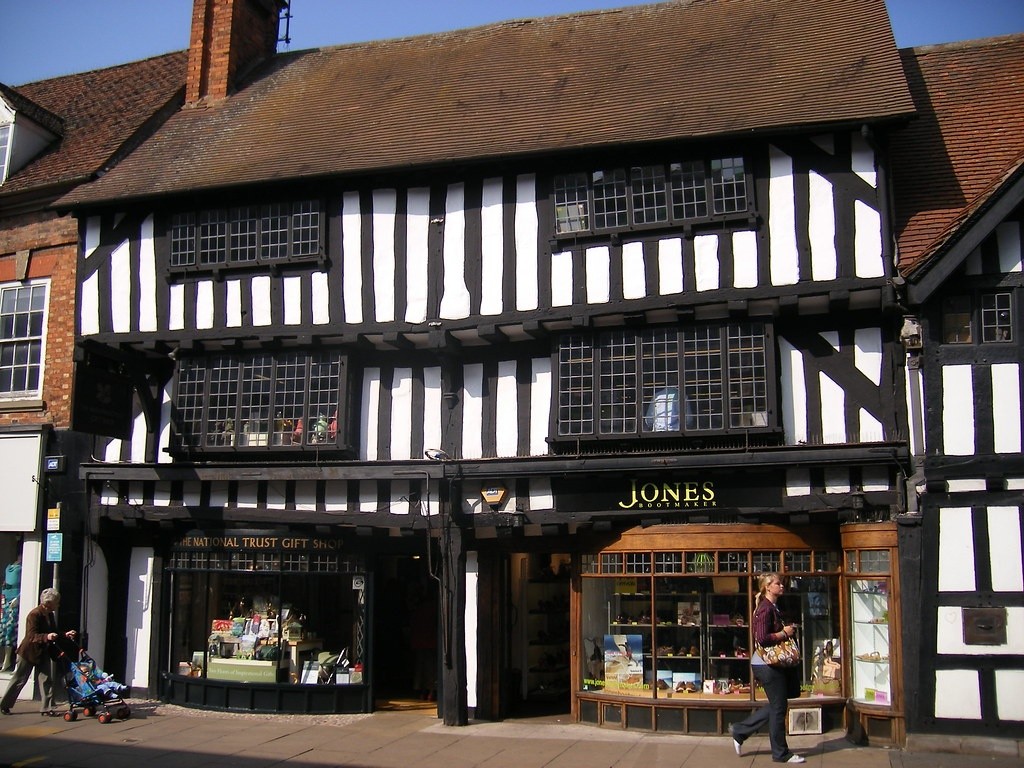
[208,638,323,683]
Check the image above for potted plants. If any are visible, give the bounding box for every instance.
[799,684,812,698]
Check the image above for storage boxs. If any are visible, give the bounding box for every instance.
[645,670,702,692]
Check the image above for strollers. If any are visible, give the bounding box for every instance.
[47,634,132,724]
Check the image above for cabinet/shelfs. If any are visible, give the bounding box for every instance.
[608,591,811,690]
[851,579,892,707]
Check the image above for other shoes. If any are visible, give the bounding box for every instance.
[728,725,743,757]
[787,753,805,764]
[0,705,12,716]
[40,707,58,713]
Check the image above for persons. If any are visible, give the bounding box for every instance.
[74,665,131,699]
[0,550,22,674]
[727,572,805,763]
[645,372,693,432]
[822,641,841,679]
[0,587,77,716]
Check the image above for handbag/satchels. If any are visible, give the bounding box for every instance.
[754,628,801,669]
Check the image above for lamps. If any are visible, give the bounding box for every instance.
[168,346,180,361]
[512,505,526,527]
[850,486,866,509]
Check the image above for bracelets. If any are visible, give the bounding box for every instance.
[780,630,787,638]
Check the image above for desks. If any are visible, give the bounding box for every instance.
[211,658,289,671]
